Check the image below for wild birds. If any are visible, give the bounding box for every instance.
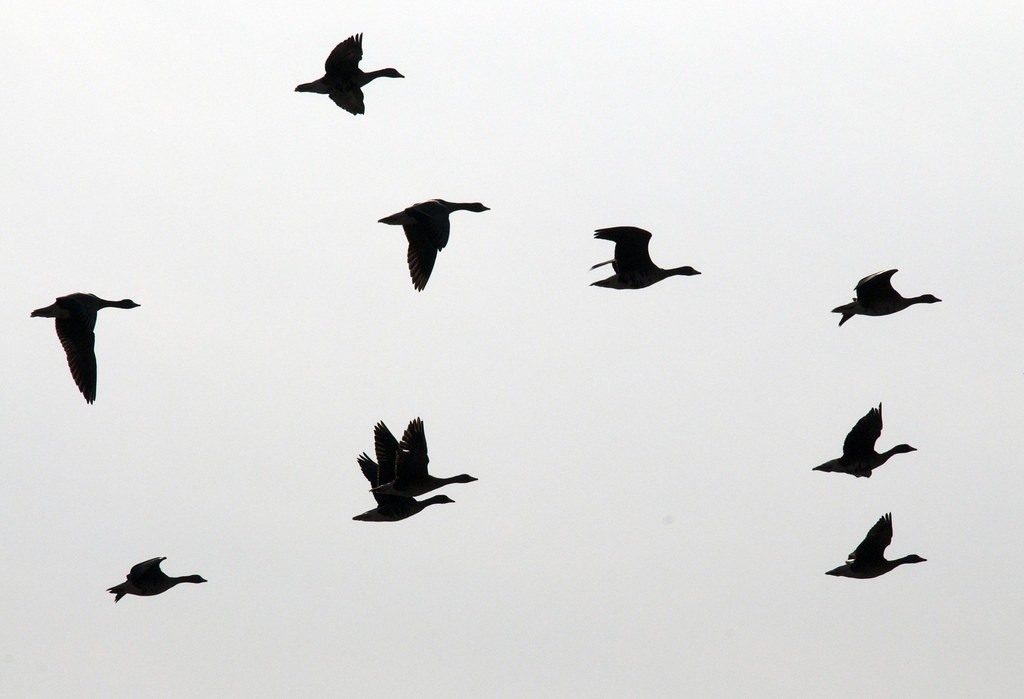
[30,292,141,405]
[353,418,480,522]
[106,556,208,603]
[811,402,917,479]
[588,226,702,291]
[831,268,942,327]
[377,198,491,292]
[826,511,929,579]
[294,32,405,116]
